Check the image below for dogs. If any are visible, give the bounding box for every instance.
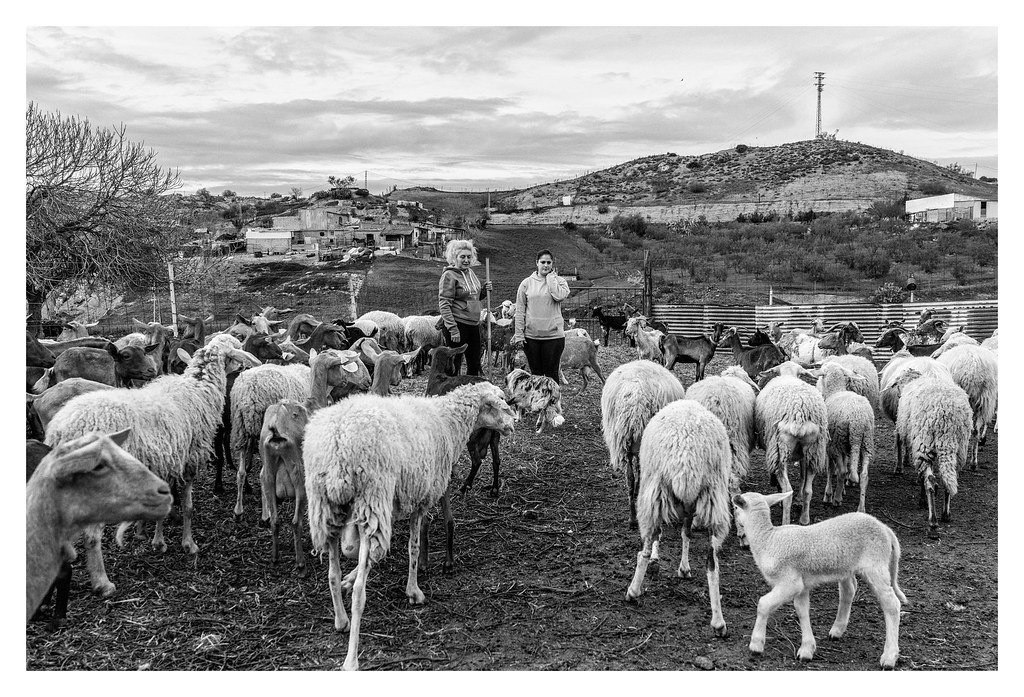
[503,364,566,436]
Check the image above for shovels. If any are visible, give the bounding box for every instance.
[484,254,494,386]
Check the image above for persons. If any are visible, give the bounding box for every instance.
[435,239,492,376]
[515,248,570,386]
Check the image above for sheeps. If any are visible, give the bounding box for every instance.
[26,303,472,671]
[729,489,910,670]
[477,300,606,399]
[302,383,515,671]
[591,302,998,671]
